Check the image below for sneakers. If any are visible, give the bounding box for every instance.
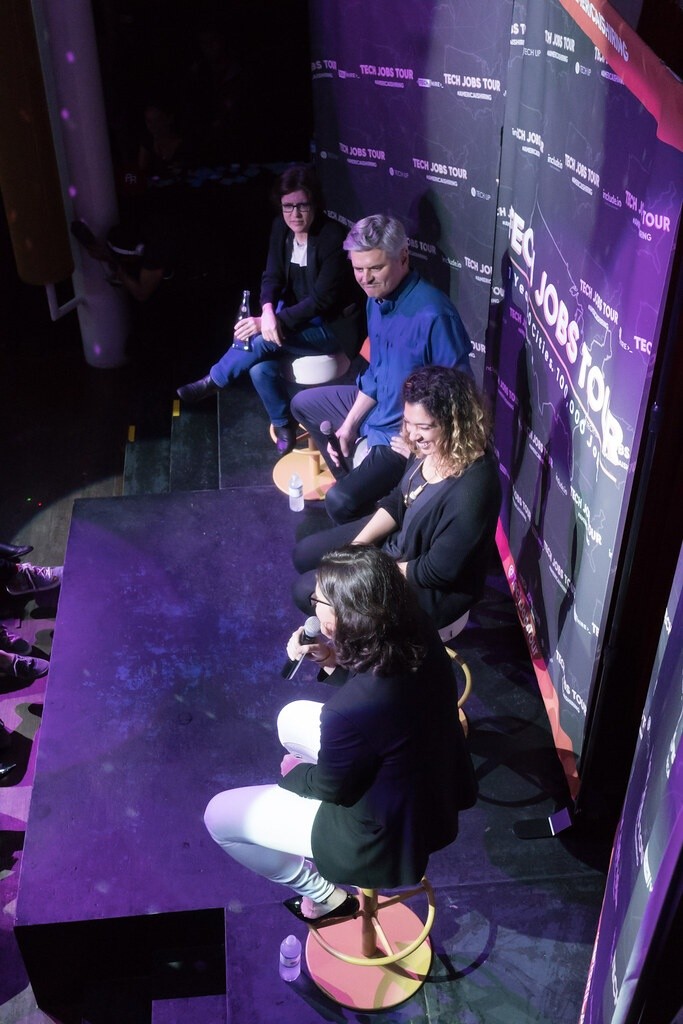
[0,623,24,648]
[0,653,51,679]
[6,560,65,595]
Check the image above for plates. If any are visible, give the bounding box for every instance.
[278,935,301,981]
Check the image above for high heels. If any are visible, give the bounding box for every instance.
[282,890,360,925]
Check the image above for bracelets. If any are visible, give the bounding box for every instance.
[315,647,331,662]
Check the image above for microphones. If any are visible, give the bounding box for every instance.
[280,616,322,680]
[320,420,350,473]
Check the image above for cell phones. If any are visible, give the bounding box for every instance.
[343,303,356,318]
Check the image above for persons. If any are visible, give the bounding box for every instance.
[290,368,502,617]
[204,544,479,925]
[291,215,473,526]
[0,542,64,775]
[176,167,351,454]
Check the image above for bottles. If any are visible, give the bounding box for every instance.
[288,474,304,512]
[232,290,250,351]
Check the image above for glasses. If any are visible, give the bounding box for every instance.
[308,590,332,608]
[282,203,309,212]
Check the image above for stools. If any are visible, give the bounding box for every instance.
[305,611,472,1011]
[268,354,354,501]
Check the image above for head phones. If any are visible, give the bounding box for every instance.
[102,238,146,256]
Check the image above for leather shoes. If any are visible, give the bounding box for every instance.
[0,542,34,558]
[276,420,299,455]
[176,373,220,402]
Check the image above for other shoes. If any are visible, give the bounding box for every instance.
[0,763,16,778]
